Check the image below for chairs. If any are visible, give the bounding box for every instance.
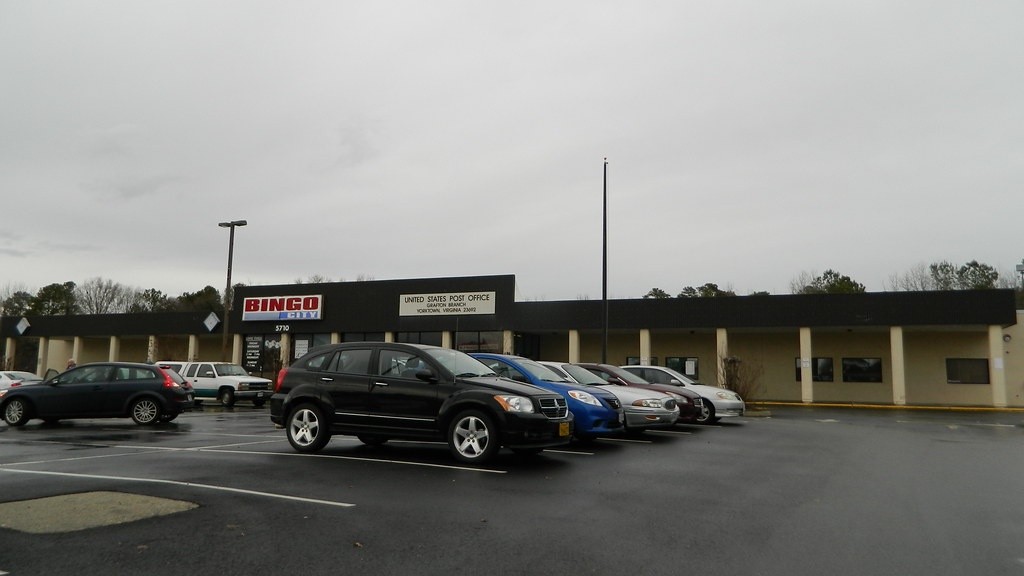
[116,369,124,379]
[400,357,419,378]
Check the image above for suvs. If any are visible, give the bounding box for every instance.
[270,340,576,466]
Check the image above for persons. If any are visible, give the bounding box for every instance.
[65,359,79,383]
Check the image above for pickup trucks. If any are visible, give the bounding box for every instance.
[146,361,274,410]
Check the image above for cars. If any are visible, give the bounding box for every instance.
[571,361,705,423]
[405,351,627,448]
[0,360,196,425]
[0,371,45,397]
[487,361,682,438]
[615,365,746,426]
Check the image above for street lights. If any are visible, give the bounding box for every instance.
[216,218,248,361]
